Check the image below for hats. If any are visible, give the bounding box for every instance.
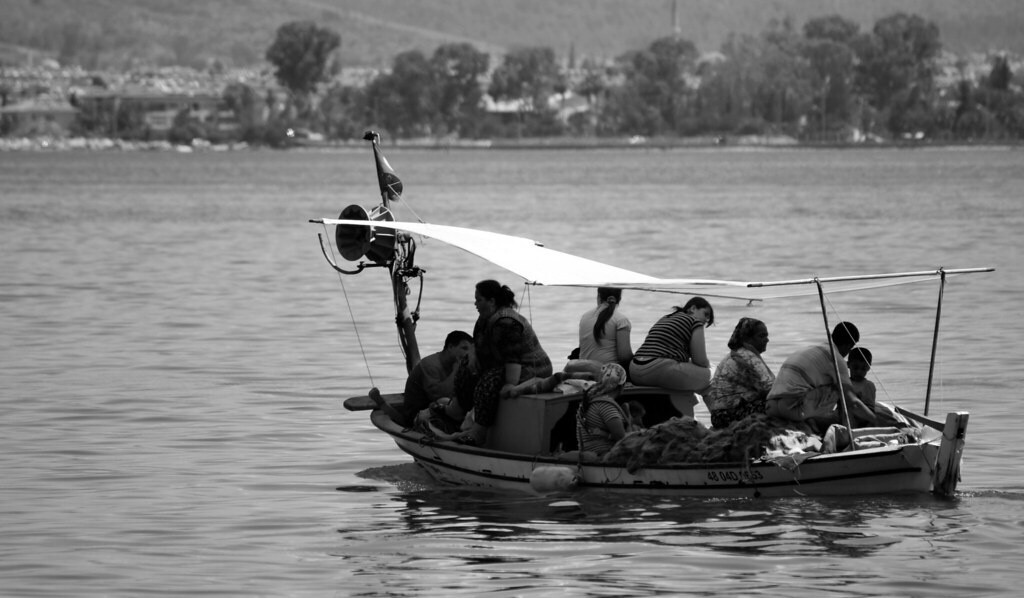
[600,363,626,390]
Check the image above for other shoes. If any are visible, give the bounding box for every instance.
[457,431,486,445]
[430,402,457,433]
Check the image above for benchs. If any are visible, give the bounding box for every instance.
[492,383,694,454]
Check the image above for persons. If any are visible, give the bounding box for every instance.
[703,316,775,430]
[846,348,876,426]
[404,278,634,457]
[766,322,878,431]
[629,296,716,415]
